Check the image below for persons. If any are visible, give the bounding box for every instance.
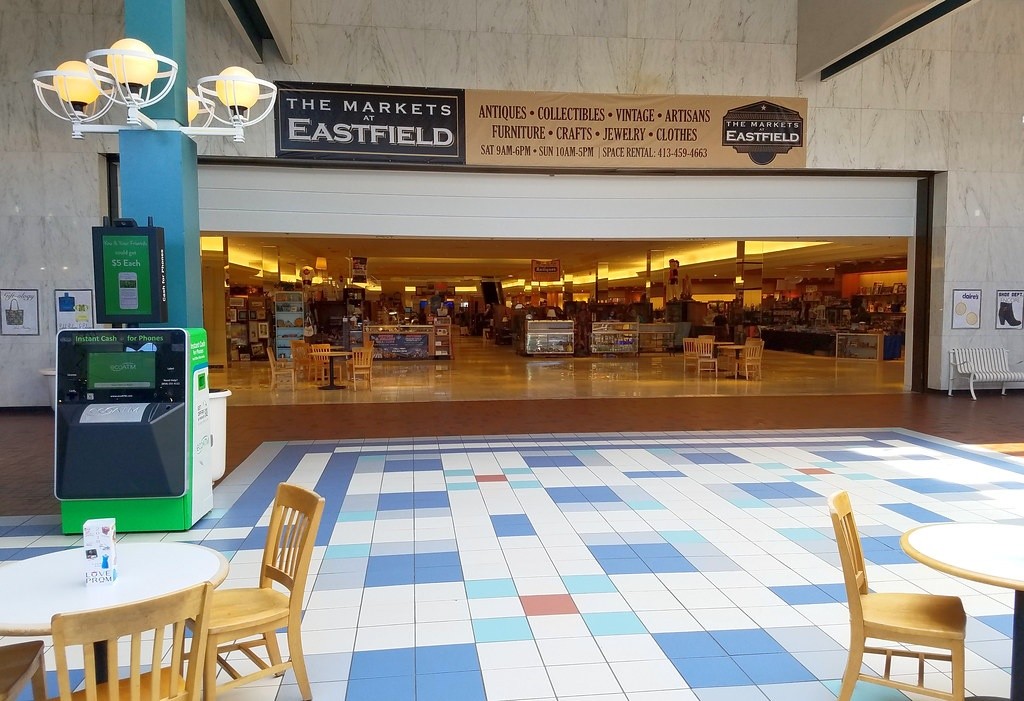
[484,304,494,319]
[798,301,811,325]
[577,302,589,347]
[418,308,426,325]
[713,308,729,338]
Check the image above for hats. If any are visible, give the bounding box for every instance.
[502,317,508,322]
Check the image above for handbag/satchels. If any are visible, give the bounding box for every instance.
[304,317,314,336]
[5,299,24,325]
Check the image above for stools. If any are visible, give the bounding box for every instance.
[696,359,718,378]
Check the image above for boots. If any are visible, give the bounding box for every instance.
[999,302,1021,326]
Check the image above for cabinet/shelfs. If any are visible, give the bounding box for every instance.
[274,291,303,362]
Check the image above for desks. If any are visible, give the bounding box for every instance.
[711,342,735,372]
[307,352,353,390]
[899,521,1023,700]
[635,332,675,358]
[0,542,230,684]
[717,345,752,380]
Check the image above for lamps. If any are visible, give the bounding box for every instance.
[106,37,159,95]
[215,66,260,117]
[53,61,101,113]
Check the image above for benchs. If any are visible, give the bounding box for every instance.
[948,347,1024,401]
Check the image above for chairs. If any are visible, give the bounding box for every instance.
[290,340,315,381]
[175,483,325,701]
[51,582,213,701]
[352,346,372,391]
[267,346,298,391]
[0,640,48,701]
[683,338,700,372]
[346,341,374,380]
[825,490,966,701]
[697,339,714,373]
[698,335,715,341]
[744,338,762,360]
[735,341,765,380]
[312,344,343,383]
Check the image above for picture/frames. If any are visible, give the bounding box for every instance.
[856,283,907,294]
[224,297,270,362]
